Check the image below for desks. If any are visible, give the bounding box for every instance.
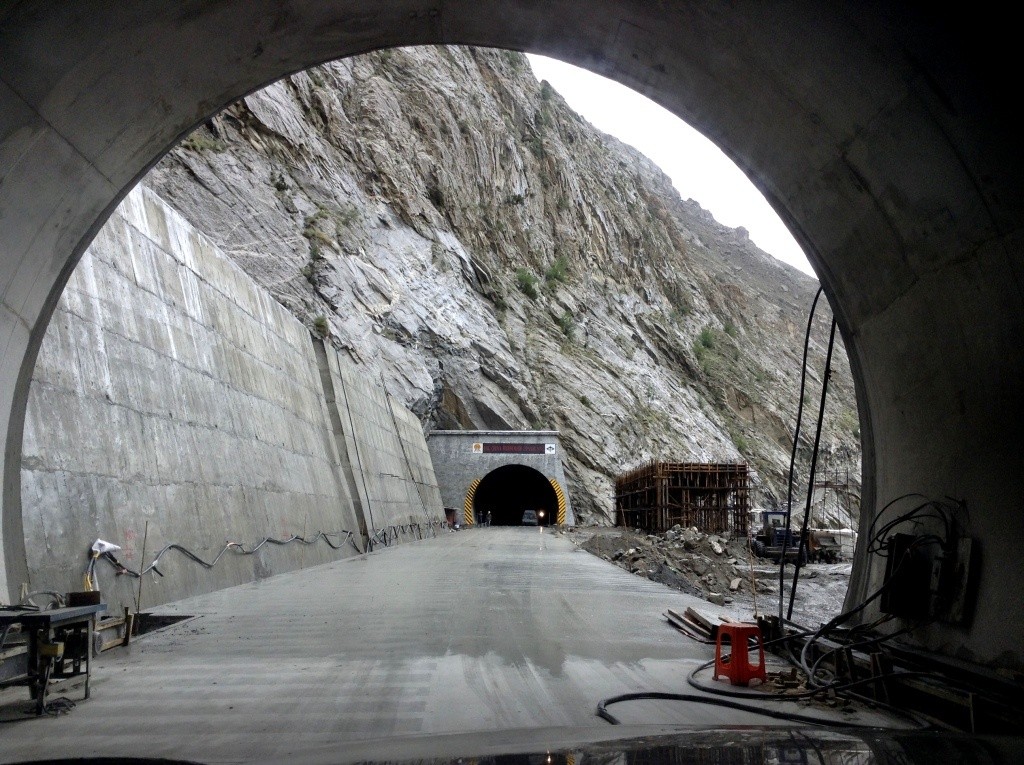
[0,604,106,716]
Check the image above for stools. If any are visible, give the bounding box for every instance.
[713,622,767,687]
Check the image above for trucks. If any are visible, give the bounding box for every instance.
[522,510,538,525]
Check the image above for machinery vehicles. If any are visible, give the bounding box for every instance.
[748,512,842,567]
